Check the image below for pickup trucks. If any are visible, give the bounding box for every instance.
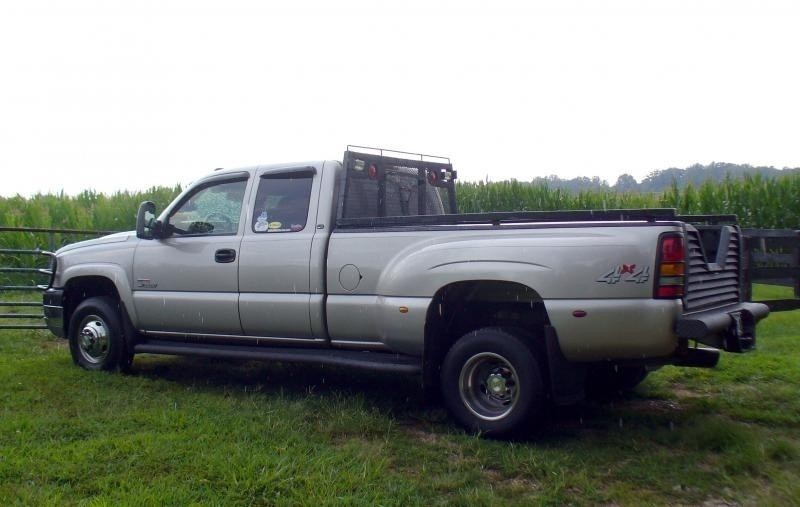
[41,143,769,441]
